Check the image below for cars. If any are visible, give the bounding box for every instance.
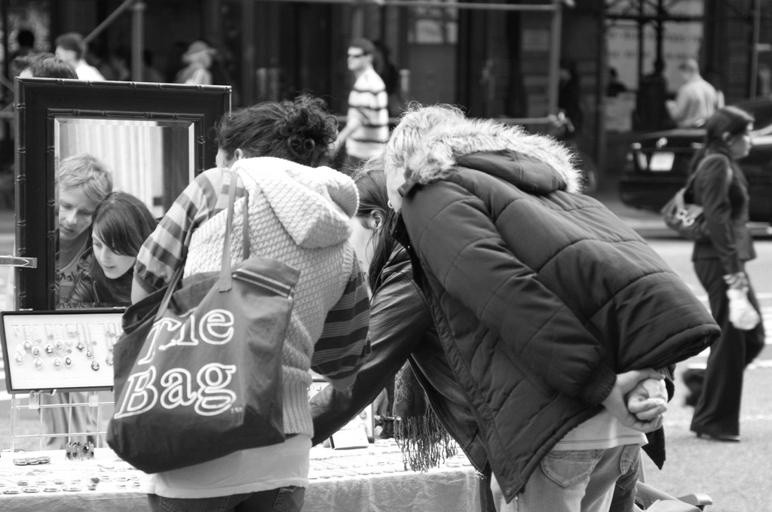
[620,94,772,222]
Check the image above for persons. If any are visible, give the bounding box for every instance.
[631,58,671,133]
[557,63,582,139]
[57,32,104,79]
[50,157,112,306]
[66,192,161,308]
[667,61,720,181]
[32,55,77,78]
[0,28,39,95]
[178,42,215,85]
[325,39,390,173]
[309,164,488,512]
[384,104,679,512]
[130,100,374,511]
[603,66,634,136]
[684,107,765,442]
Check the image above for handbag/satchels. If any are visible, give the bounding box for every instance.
[658,153,733,243]
[104,169,300,475]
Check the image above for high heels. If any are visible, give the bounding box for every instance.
[694,426,742,444]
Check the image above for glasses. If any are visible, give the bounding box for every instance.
[345,51,364,62]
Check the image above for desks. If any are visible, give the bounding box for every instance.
[0,435,481,511]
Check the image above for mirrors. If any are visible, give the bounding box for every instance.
[14,76,232,311]
